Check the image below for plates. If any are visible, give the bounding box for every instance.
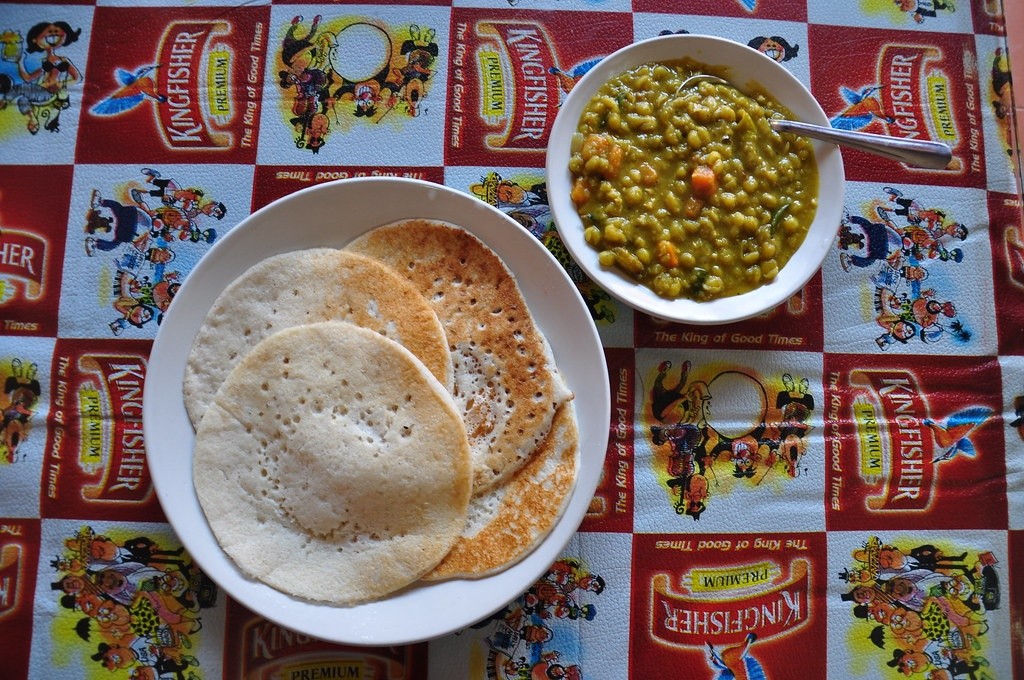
[147,176,611,646]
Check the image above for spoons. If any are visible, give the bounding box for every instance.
[677,75,953,169]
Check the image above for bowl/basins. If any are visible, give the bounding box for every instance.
[548,33,844,325]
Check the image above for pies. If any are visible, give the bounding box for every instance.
[183,217,583,607]
